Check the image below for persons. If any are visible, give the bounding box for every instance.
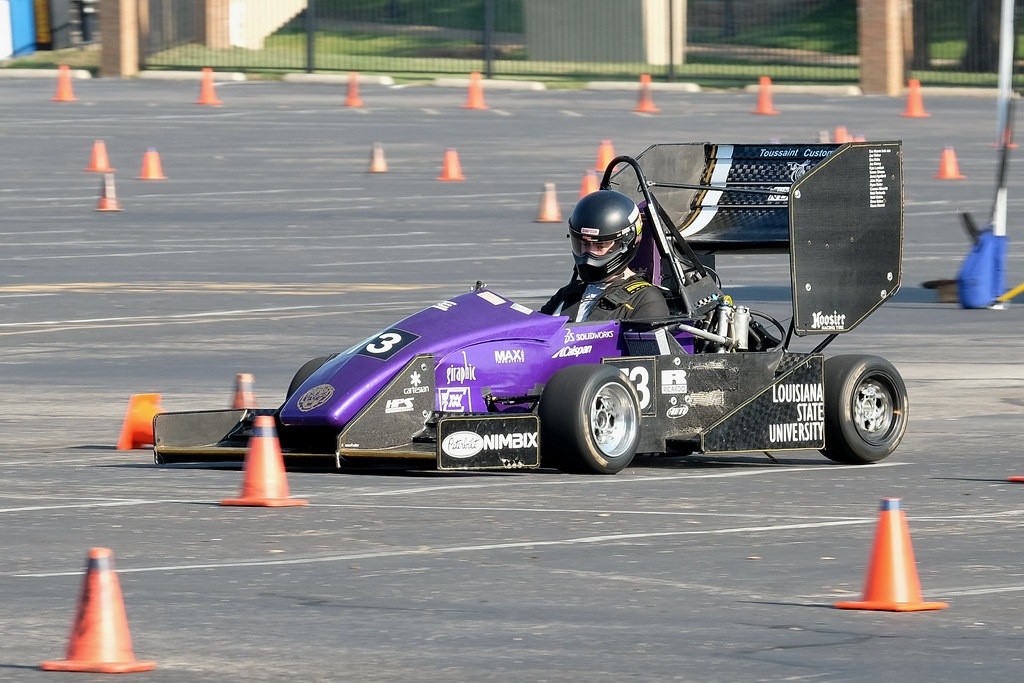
[538,190,670,323]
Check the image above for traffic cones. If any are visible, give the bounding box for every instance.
[753,76,779,115]
[900,78,930,117]
[935,148,964,180]
[632,74,659,112]
[118,394,166,449]
[234,373,255,409]
[579,169,599,199]
[368,142,389,173]
[41,547,156,673]
[195,68,222,106]
[594,140,620,174]
[833,497,950,611]
[345,73,363,106]
[220,416,310,507]
[93,147,170,212]
[533,183,564,223]
[462,72,487,109]
[434,147,465,180]
[48,65,77,101]
[82,141,114,173]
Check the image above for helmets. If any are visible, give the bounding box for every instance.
[566,190,644,285]
